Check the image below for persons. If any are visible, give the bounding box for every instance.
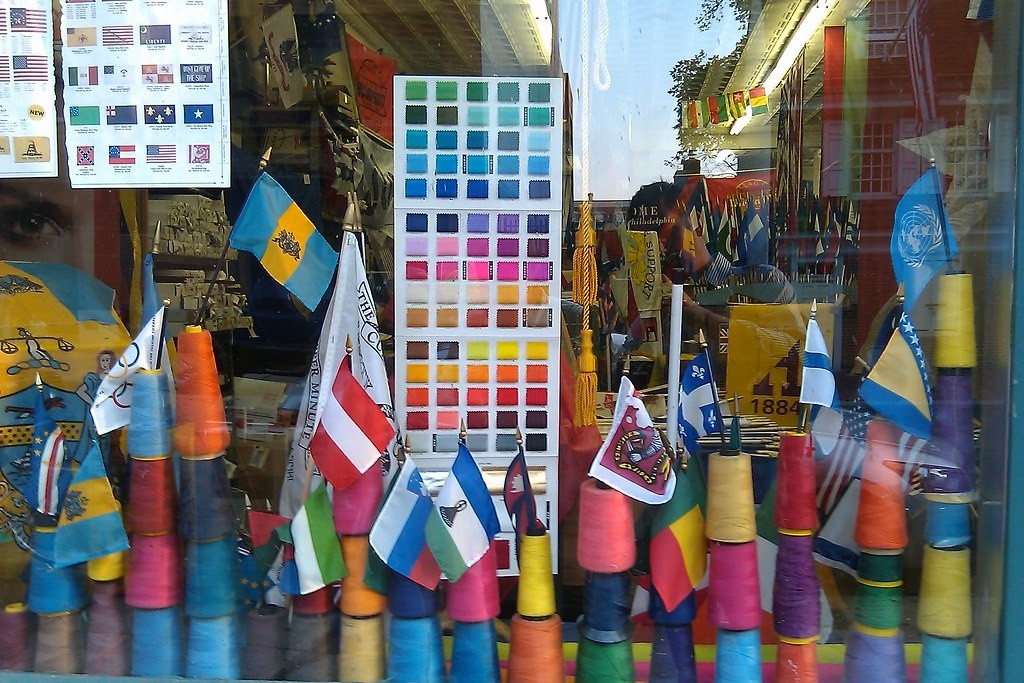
[610,182,812,506]
[1,123,96,274]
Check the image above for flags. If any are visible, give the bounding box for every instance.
[650,470,710,611]
[369,456,442,591]
[231,172,341,314]
[277,481,346,596]
[28,398,70,515]
[691,190,859,279]
[92,306,167,435]
[425,442,500,585]
[728,91,746,120]
[363,467,400,593]
[312,358,394,488]
[709,96,728,123]
[814,304,929,519]
[861,316,934,435]
[588,377,677,502]
[57,439,128,565]
[678,350,724,454]
[888,176,955,313]
[750,88,768,114]
[279,229,402,516]
[688,101,710,127]
[799,319,841,454]
